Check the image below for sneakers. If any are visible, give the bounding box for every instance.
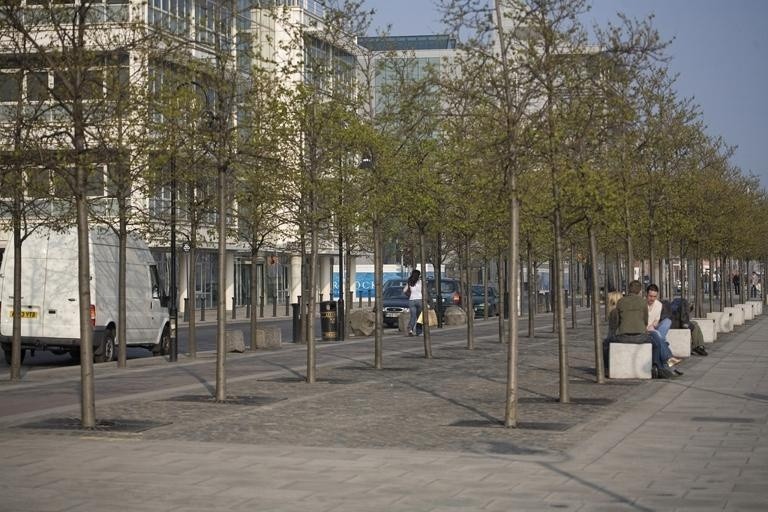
[408,330,423,336]
[659,357,683,378]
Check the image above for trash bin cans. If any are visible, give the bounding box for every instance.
[291,303,310,343]
[564,290,568,308]
[320,301,338,341]
[545,291,553,312]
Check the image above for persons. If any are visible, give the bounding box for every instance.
[603,280,681,379]
[713,271,720,299]
[751,271,758,298]
[670,298,708,356]
[403,270,422,336]
[734,272,740,294]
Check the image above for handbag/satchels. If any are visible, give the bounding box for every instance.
[416,309,438,326]
[404,286,411,297]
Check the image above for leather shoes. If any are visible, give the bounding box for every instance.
[695,345,708,356]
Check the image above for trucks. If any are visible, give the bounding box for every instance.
[0,217,176,366]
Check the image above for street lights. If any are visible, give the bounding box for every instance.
[165,79,215,363]
[337,142,376,340]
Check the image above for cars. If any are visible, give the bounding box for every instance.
[676,279,681,292]
[374,275,499,328]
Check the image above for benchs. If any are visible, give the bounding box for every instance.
[609,298,765,379]
